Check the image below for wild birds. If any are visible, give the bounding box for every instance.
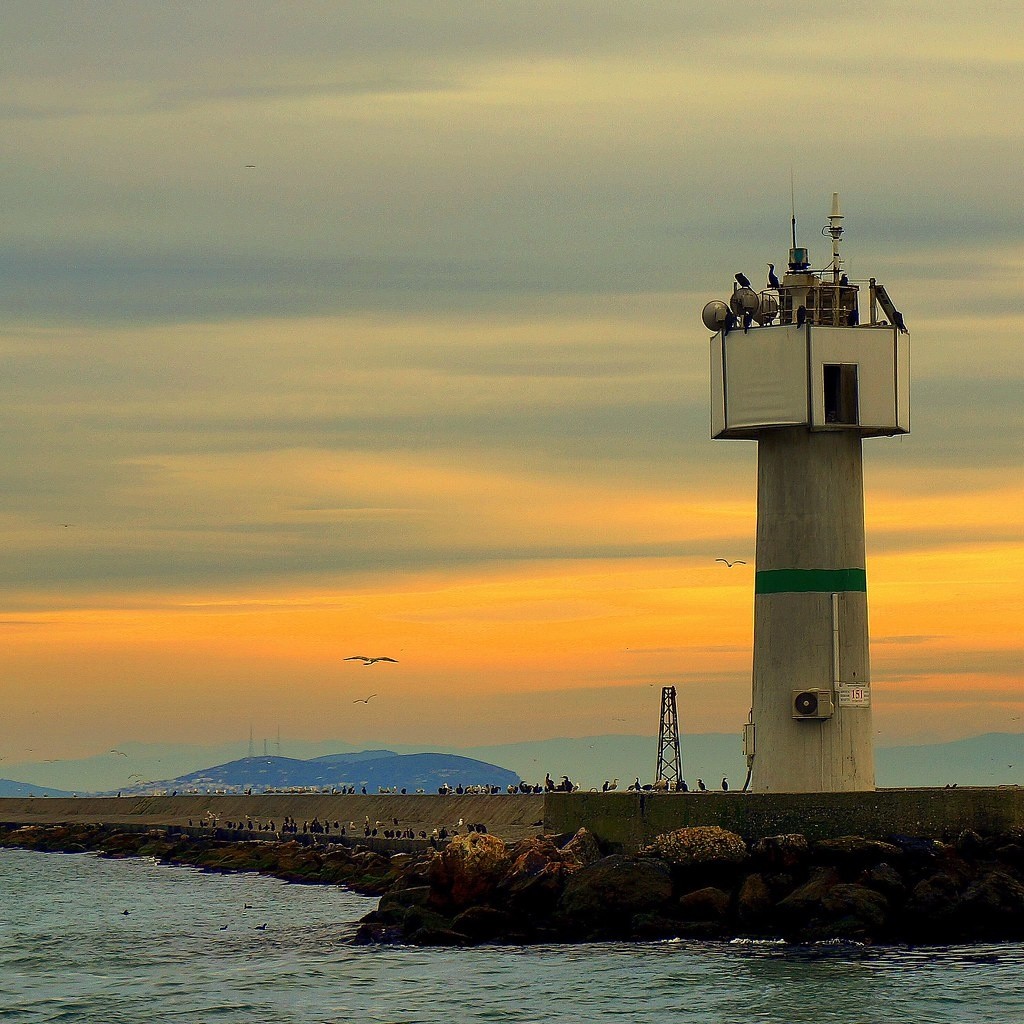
[715,558,746,567]
[723,272,806,335]
[839,274,905,335]
[767,263,780,288]
[0,655,728,939]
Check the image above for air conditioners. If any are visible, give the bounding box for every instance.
[792,689,834,718]
